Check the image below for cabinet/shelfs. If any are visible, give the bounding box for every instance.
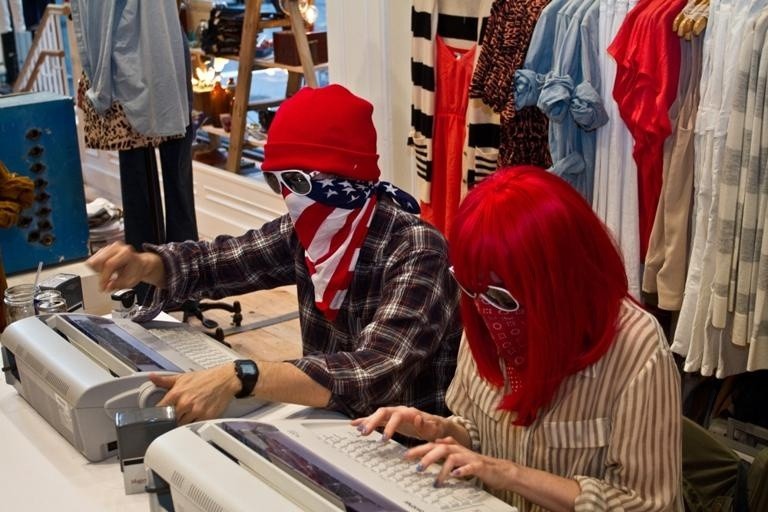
[189,47,328,148]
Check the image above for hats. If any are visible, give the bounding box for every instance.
[260,82,382,182]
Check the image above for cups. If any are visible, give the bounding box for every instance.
[4,282,41,324]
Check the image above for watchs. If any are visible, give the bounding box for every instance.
[230,354,259,403]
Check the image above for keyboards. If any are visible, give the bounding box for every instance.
[146,320,236,374]
[311,420,520,512]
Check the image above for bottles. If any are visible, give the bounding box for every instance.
[212,77,235,128]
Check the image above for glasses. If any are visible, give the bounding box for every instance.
[447,265,523,314]
[259,168,324,196]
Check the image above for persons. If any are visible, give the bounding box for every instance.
[73,2,201,246]
[77,78,461,434]
[347,164,692,510]
